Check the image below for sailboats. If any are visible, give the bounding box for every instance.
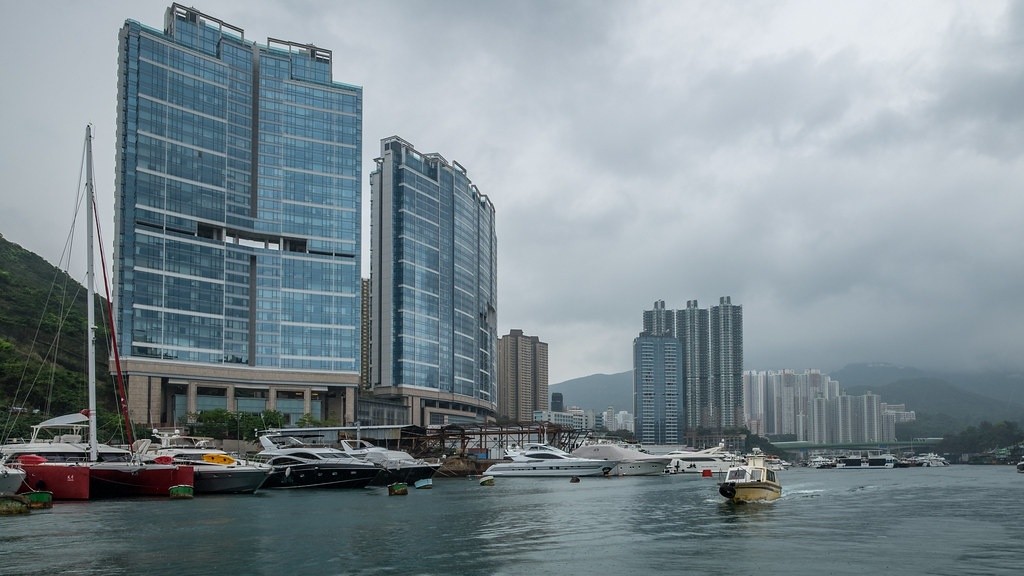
[1,122,152,502]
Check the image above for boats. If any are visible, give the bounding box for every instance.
[802,450,952,471]
[150,445,274,496]
[245,434,384,488]
[658,444,794,475]
[337,438,441,486]
[0,459,27,497]
[479,442,620,479]
[569,445,673,479]
[716,451,783,505]
